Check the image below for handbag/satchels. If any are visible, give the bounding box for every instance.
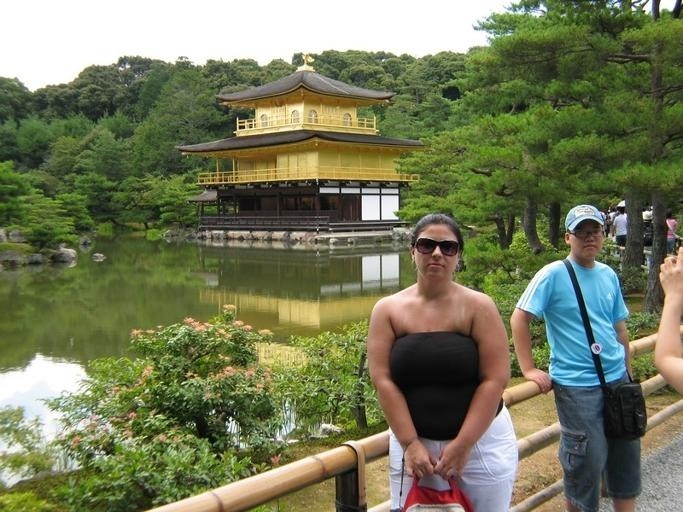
[401,465,474,511]
[605,379,647,443]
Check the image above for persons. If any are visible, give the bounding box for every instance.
[594,197,679,256]
[509,203,642,512]
[366,212,520,512]
[651,245,682,396]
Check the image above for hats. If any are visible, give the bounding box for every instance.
[565,204,605,233]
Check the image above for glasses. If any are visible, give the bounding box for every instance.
[416,238,461,257]
[568,225,604,239]
